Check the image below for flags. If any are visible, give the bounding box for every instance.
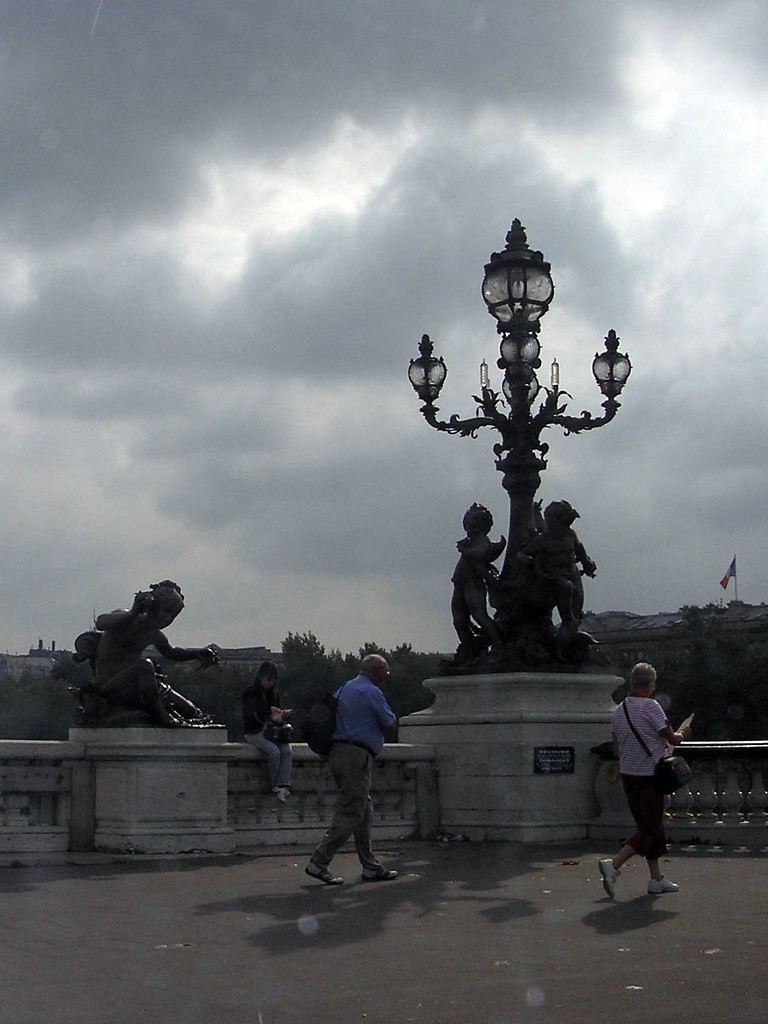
[720,558,736,590]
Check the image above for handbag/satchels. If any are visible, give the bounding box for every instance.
[653,754,693,795]
[268,721,294,743]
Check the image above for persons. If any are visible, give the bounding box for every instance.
[305,654,399,885]
[598,662,692,898]
[72,580,220,728]
[450,503,506,663]
[242,661,293,804]
[517,499,597,662]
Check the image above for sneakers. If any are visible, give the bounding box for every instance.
[272,785,290,804]
[360,865,398,882]
[598,858,621,899]
[305,860,345,885]
[647,873,679,893]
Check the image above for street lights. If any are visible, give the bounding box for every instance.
[408,215,633,674]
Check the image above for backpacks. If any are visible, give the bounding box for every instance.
[301,679,350,761]
